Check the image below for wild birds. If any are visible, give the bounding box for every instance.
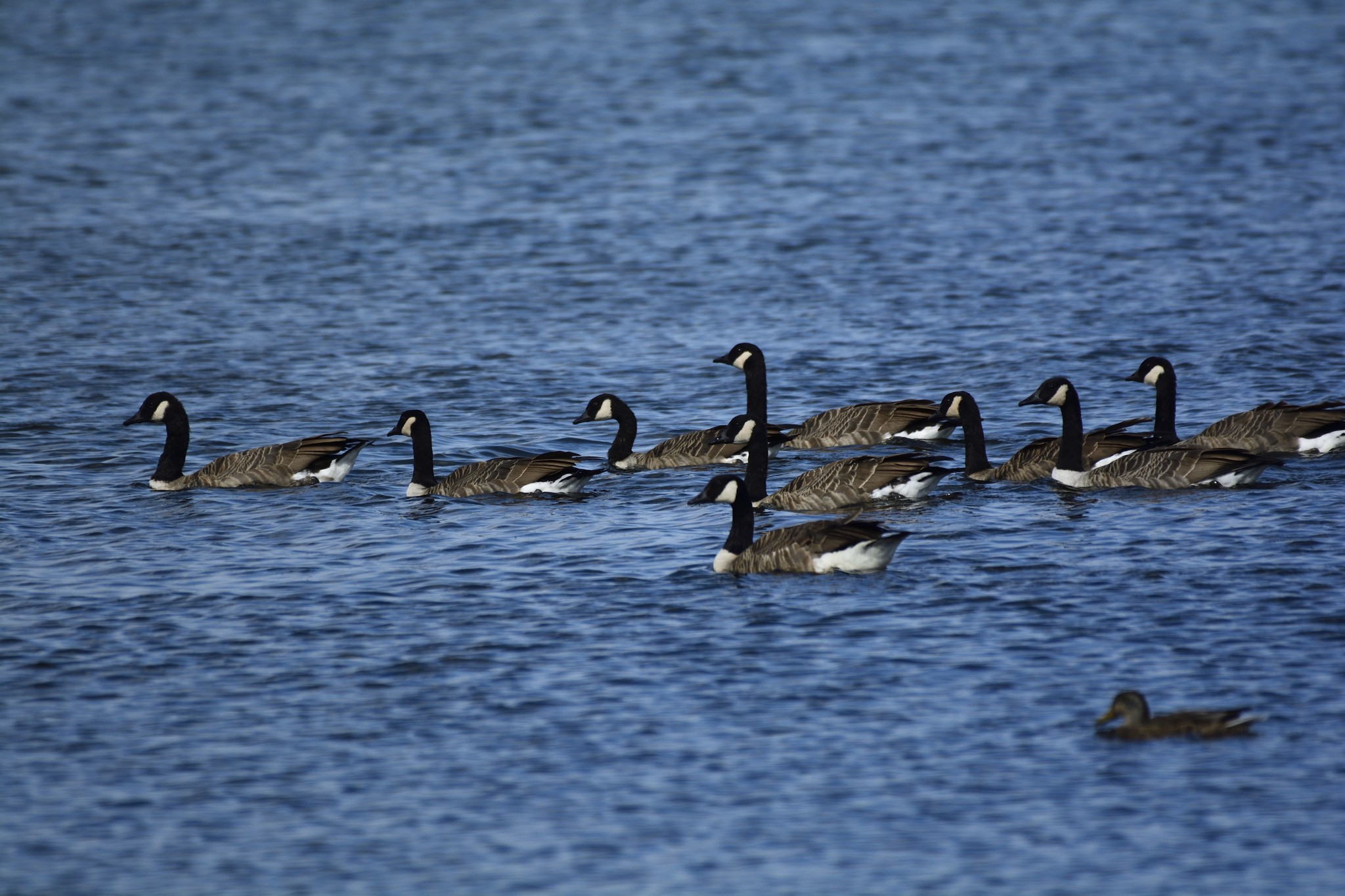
[1113,357,1345,458]
[387,409,606,499]
[710,416,954,516]
[1017,377,1297,489]
[124,391,377,491]
[572,393,802,474]
[1095,690,1256,738]
[687,473,915,576]
[712,343,955,450]
[930,390,1158,486]
